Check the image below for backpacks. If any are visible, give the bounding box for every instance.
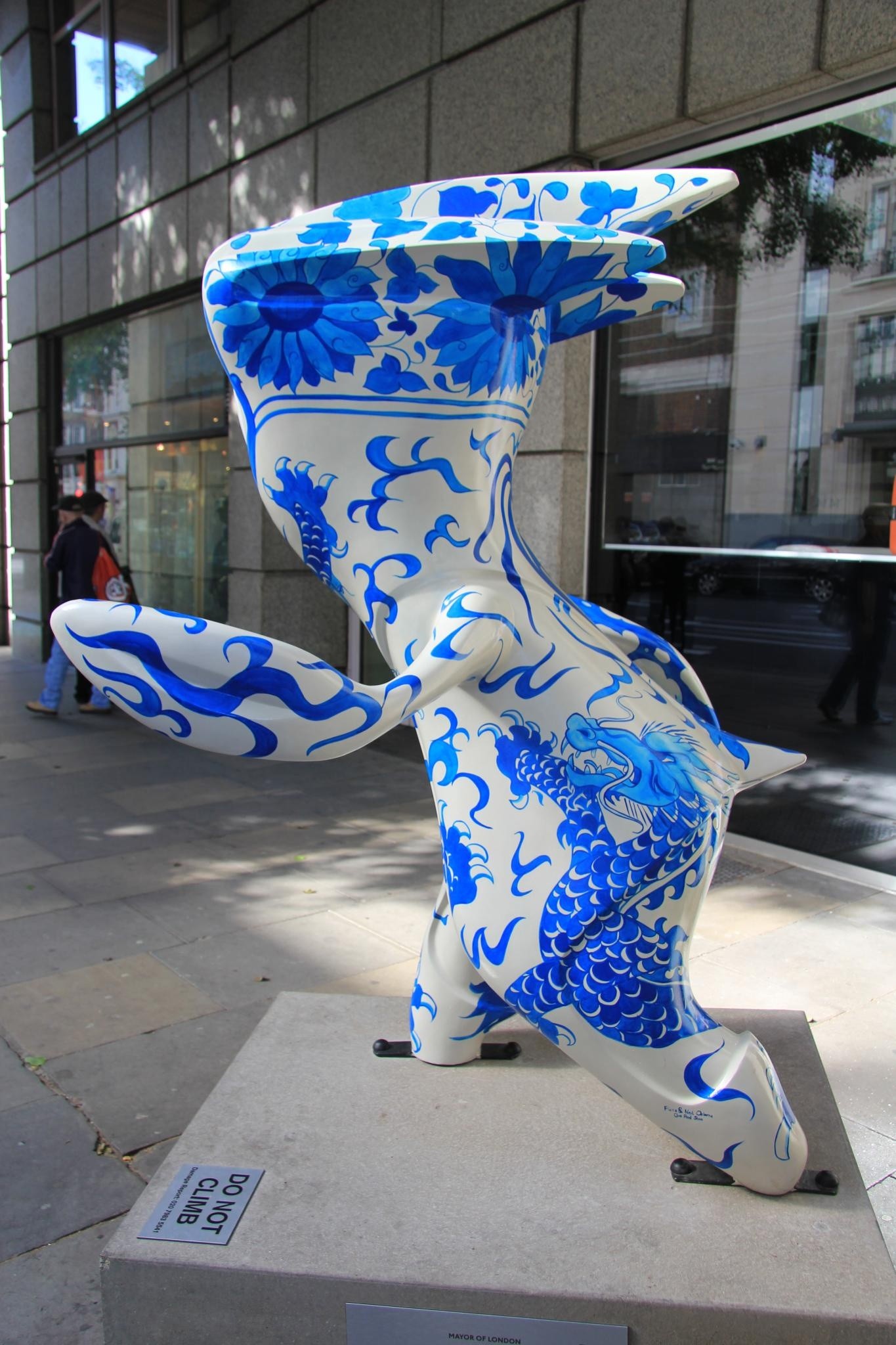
[92,531,132,604]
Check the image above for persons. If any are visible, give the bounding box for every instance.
[644,516,688,656]
[817,502,893,727]
[25,492,139,715]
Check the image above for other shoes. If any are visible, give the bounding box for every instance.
[79,702,105,712]
[26,700,58,715]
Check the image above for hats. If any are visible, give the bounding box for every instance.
[81,492,108,506]
[51,495,83,511]
